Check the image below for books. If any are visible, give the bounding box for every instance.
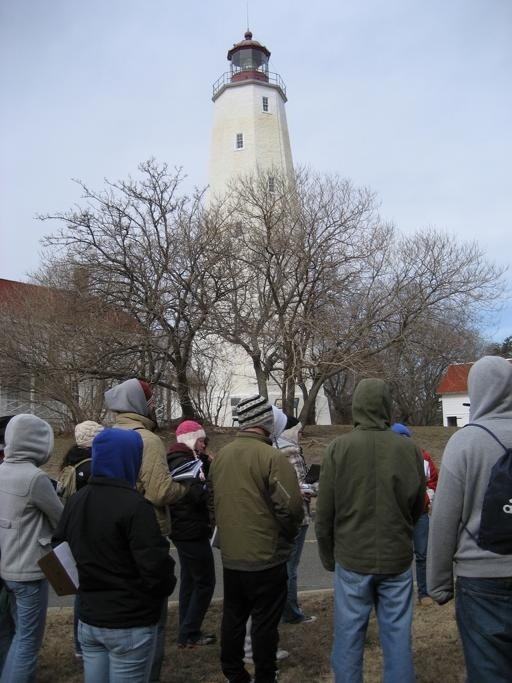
[211,525,221,549]
[169,458,203,481]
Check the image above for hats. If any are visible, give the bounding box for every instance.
[140,380,153,401]
[74,421,105,449]
[279,415,302,443]
[235,393,274,436]
[176,420,206,450]
[391,423,411,437]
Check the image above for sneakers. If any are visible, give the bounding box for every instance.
[299,614,317,624]
[196,629,216,646]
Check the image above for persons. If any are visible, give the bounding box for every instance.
[103,377,206,537]
[270,415,317,625]
[0,412,64,683]
[51,428,170,683]
[390,422,438,607]
[56,420,106,505]
[0,415,66,502]
[426,354,512,682]
[206,392,304,683]
[166,420,218,647]
[313,377,425,683]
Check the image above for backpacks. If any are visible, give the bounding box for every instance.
[54,458,92,505]
[460,423,512,556]
